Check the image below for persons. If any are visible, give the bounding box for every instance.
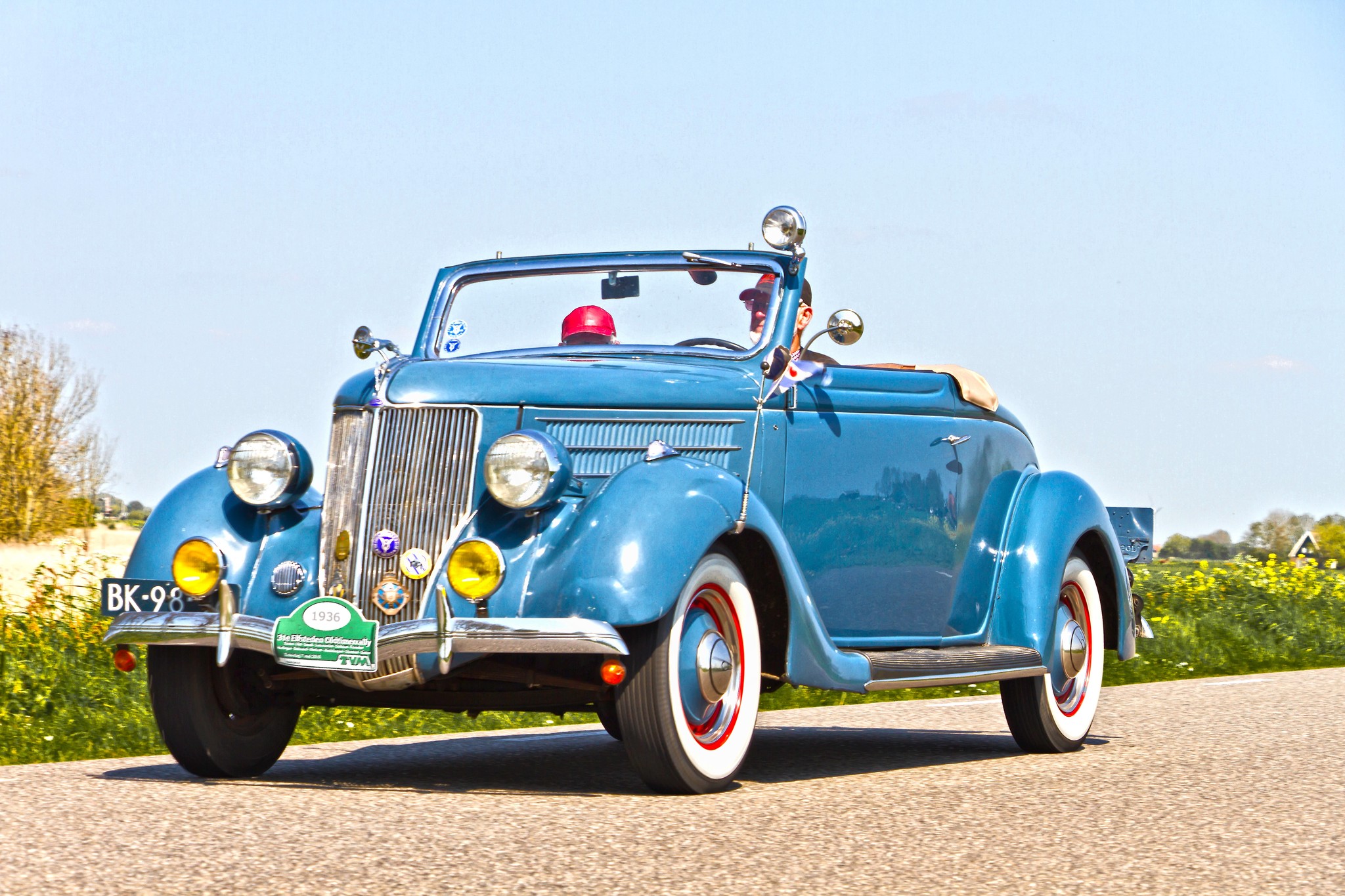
[557,305,620,347]
[738,271,841,366]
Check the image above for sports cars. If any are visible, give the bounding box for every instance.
[99,206,1156,795]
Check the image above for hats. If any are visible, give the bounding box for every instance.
[562,305,617,341]
[739,274,812,308]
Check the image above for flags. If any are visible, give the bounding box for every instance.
[773,361,824,397]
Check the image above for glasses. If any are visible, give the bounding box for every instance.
[743,299,782,313]
[563,341,616,345]
